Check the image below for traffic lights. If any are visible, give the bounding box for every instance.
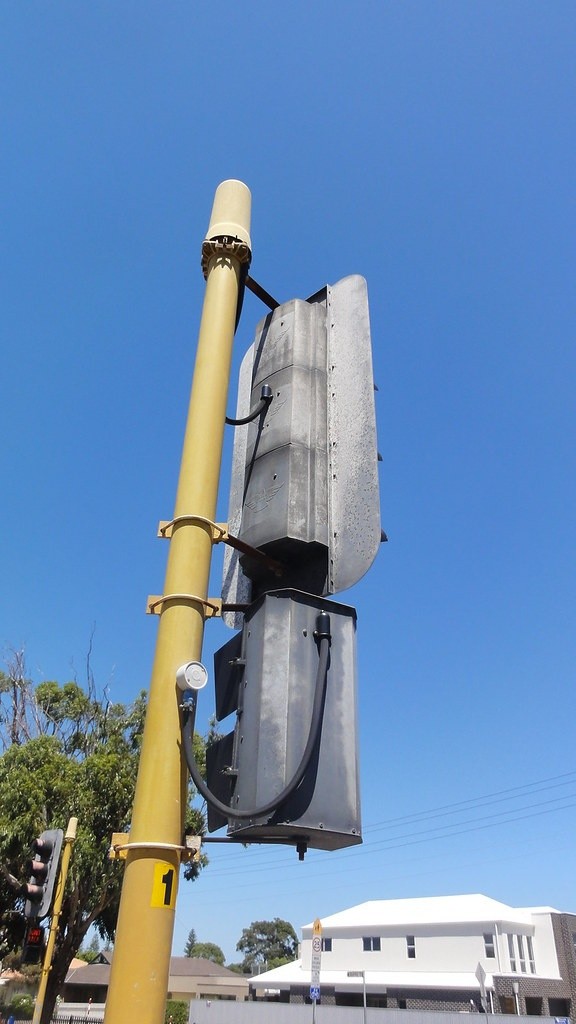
[23,926,46,963]
[20,838,54,901]
